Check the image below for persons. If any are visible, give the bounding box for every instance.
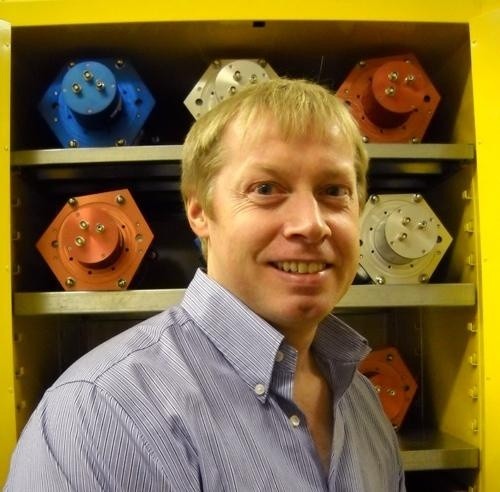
[0,75,405,491]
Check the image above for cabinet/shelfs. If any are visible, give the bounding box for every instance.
[1,0,500,491]
